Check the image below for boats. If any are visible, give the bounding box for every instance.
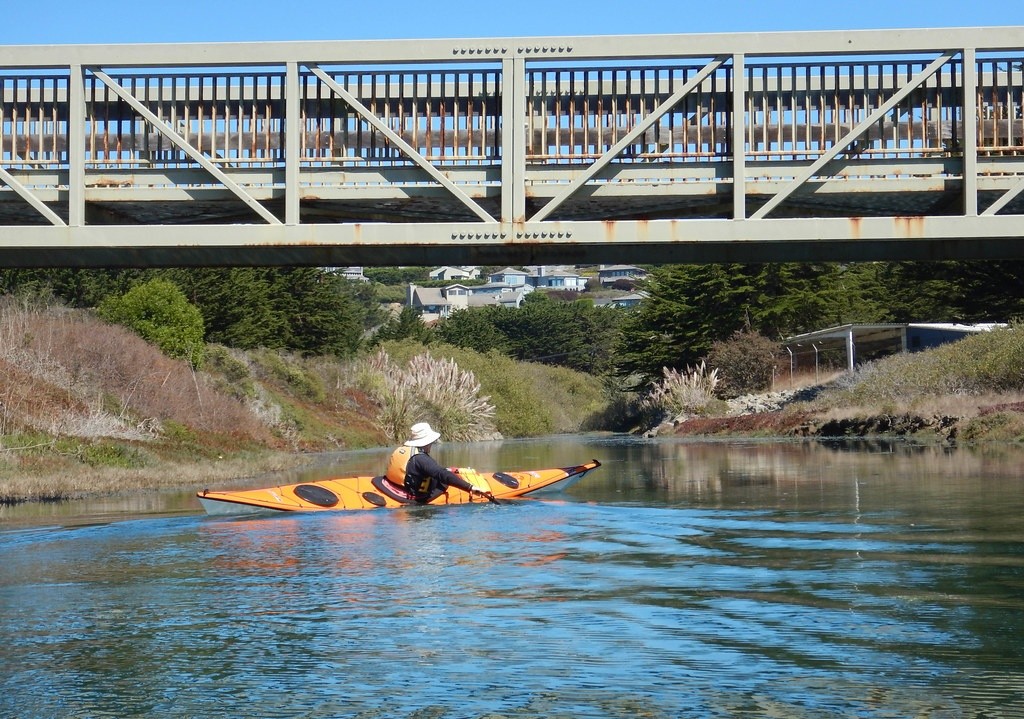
[197,459,603,515]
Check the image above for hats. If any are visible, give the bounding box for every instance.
[403,423,441,448]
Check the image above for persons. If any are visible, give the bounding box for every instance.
[384,421,491,503]
